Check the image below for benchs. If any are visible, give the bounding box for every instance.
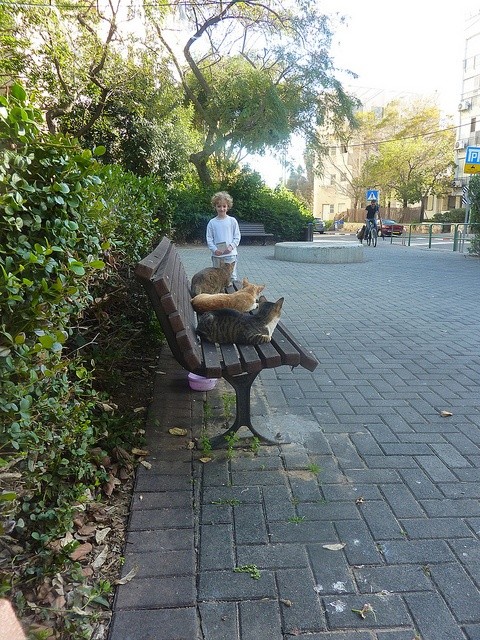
[239,224,274,246]
[133,235,319,451]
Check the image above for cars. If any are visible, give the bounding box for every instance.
[376,219,404,236]
[312,218,325,234]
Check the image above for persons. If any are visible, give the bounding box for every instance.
[364,200,381,240]
[206,191,241,280]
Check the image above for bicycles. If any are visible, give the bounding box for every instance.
[366,221,379,247]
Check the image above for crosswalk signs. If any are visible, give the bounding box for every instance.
[366,190,379,201]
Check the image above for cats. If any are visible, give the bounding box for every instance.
[191,277,266,312]
[195,295,285,344]
[190,259,236,299]
